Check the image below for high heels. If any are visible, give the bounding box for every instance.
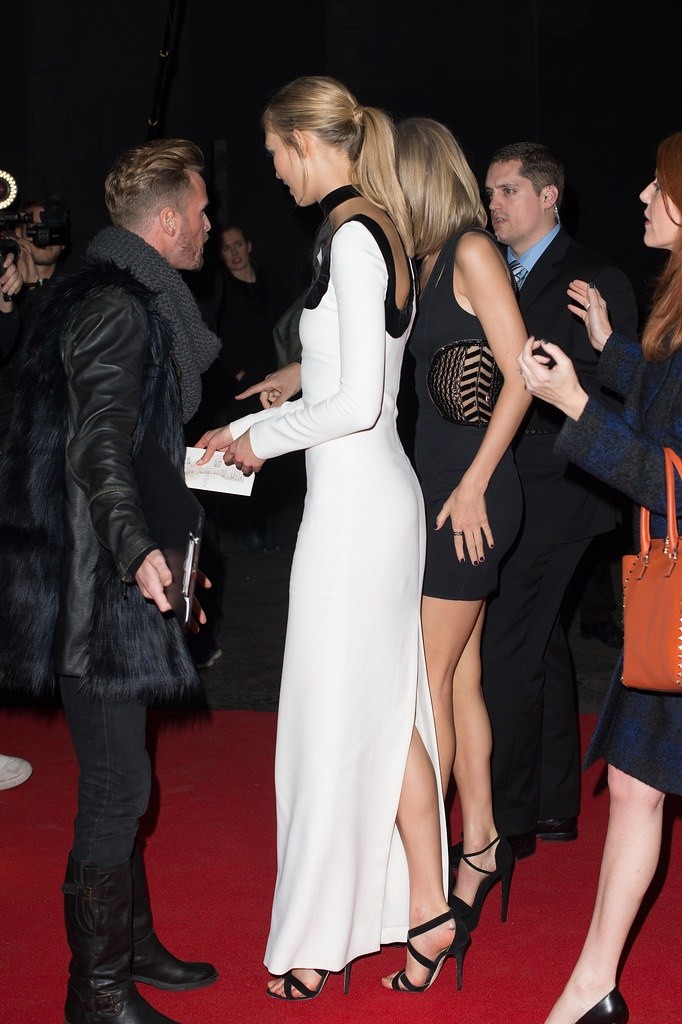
[267,961,351,1000]
[450,834,516,929]
[382,908,469,992]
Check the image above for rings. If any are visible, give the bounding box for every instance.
[585,304,591,309]
[520,370,522,375]
[453,532,463,536]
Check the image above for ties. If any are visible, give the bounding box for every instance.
[509,260,528,292]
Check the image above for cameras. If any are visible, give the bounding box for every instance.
[0,211,34,302]
[22,194,64,269]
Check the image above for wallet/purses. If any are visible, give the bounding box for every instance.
[185,446,255,498]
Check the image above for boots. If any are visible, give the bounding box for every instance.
[62,851,180,1024]
[130,856,219,990]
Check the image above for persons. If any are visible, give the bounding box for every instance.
[0,139,222,1024]
[1,195,66,791]
[192,222,291,552]
[193,75,471,1002]
[397,116,533,931]
[518,132,682,1024]
[448,141,637,869]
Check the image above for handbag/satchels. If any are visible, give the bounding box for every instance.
[620,448,682,693]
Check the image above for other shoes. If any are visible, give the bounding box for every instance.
[238,527,266,553]
[574,986,628,1024]
[0,754,32,790]
[183,633,223,668]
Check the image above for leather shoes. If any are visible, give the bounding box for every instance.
[449,831,536,862]
[534,815,578,842]
